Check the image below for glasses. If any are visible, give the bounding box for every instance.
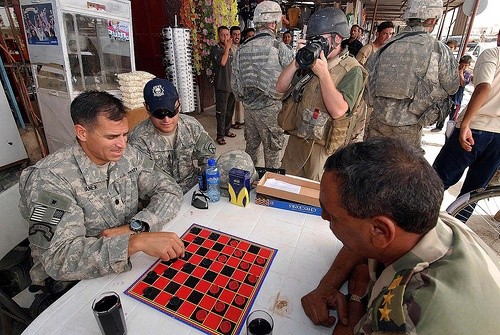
[191,190,211,209]
[150,104,180,119]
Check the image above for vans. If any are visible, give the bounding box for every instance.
[439,35,481,52]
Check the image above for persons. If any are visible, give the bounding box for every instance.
[21,6,57,43]
[128,77,217,195]
[18,91,185,301]
[447,39,457,51]
[211,25,256,144]
[229,1,295,168]
[480,30,487,43]
[300,135,500,335]
[361,0,459,156]
[275,7,369,182]
[430,29,500,226]
[282,30,292,45]
[431,54,473,133]
[341,21,396,68]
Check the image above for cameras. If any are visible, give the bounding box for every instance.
[295,35,330,69]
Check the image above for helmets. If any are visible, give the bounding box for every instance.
[306,7,350,37]
[215,149,259,197]
[403,0,443,19]
[253,1,282,22]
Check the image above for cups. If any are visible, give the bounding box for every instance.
[91,291,128,335]
[246,310,274,335]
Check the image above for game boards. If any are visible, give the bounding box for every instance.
[123,222,278,335]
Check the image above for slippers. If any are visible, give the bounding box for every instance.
[217,137,226,145]
[225,131,236,137]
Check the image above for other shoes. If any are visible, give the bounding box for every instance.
[231,124,241,129]
[431,127,442,132]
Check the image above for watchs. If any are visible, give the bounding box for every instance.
[127,219,147,234]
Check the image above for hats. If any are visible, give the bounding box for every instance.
[143,78,179,112]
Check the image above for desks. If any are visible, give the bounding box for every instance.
[20,182,348,335]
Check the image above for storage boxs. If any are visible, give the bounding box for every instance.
[256,171,323,216]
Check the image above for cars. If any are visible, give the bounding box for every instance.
[107,19,130,41]
[467,42,497,63]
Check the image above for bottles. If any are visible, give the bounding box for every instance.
[205,159,221,203]
[445,120,455,139]
[198,163,208,193]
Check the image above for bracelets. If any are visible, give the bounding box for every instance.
[349,293,362,303]
